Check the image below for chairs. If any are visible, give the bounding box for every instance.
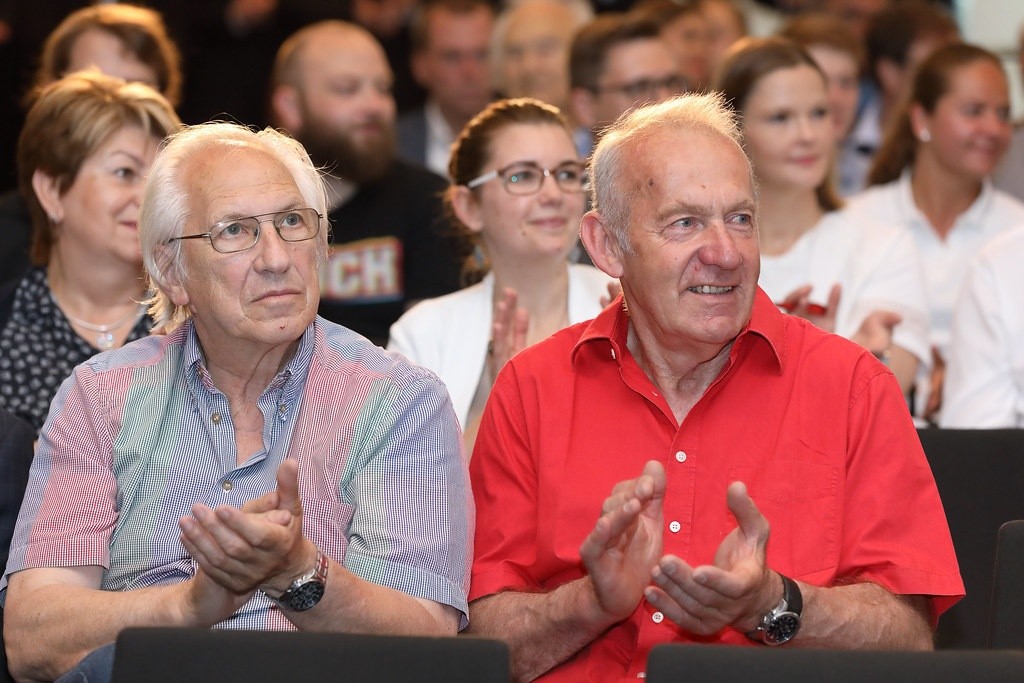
[114,431,1024,683]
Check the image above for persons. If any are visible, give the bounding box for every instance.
[0,409,38,575]
[5,121,479,682]
[0,0,1023,439]
[466,91,968,682]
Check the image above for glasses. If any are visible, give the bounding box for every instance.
[593,75,687,103]
[466,160,585,193]
[163,208,323,254]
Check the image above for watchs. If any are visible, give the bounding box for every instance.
[267,551,329,612]
[747,573,804,647]
